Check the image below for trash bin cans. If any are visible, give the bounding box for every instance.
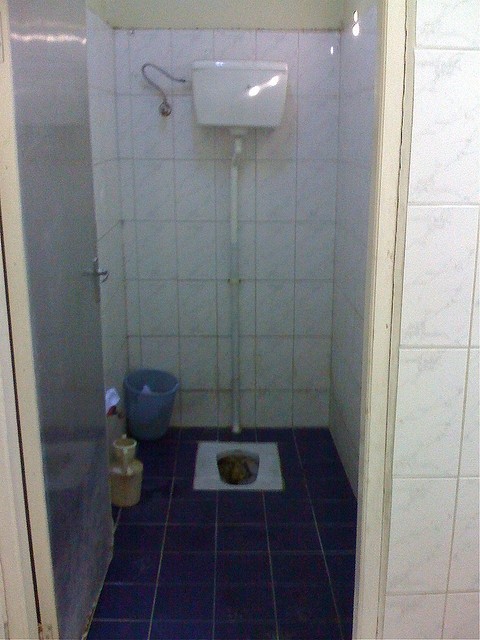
[123,370,180,441]
